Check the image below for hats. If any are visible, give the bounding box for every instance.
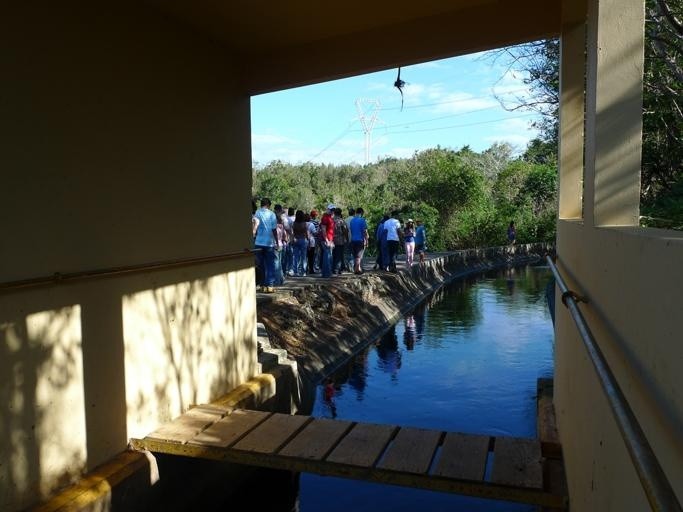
[405,219,415,225]
[310,210,319,217]
[416,218,422,222]
[328,205,336,209]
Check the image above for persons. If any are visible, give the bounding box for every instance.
[505,270,514,297]
[506,221,516,255]
[373,215,426,272]
[320,327,403,419]
[412,305,425,341]
[251,198,368,292]
[403,314,416,350]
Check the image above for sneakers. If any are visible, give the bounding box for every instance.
[256,263,413,293]
